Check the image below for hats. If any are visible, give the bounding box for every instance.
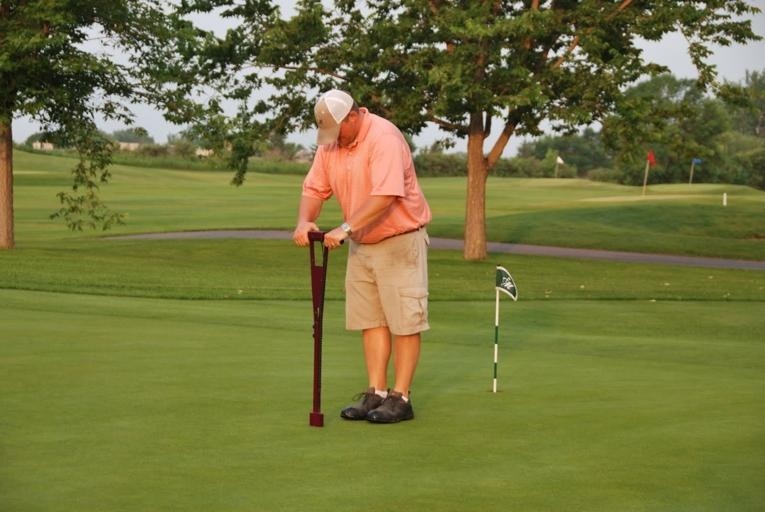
[313,89,354,145]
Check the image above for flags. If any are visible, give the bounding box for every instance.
[556,156,564,165]
[693,158,702,165]
[647,148,658,167]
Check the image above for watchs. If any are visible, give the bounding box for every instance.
[340,223,353,237]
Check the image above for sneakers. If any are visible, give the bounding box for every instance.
[340,388,413,423]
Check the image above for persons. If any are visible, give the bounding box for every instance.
[291,90,434,423]
